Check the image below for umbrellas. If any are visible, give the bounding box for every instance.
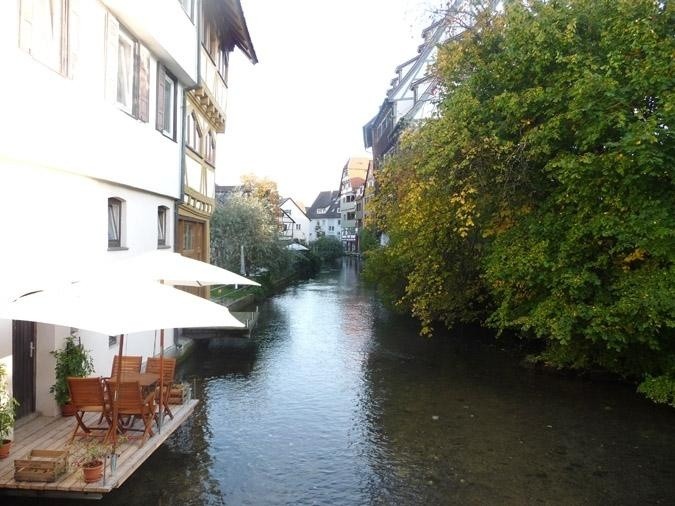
[35,242,262,421]
[0,276,245,445]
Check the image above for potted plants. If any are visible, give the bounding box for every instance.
[1,362,20,459]
[50,331,97,417]
[156,381,194,405]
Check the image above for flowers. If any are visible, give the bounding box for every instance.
[62,429,136,484]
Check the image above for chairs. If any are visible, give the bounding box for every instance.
[65,352,177,448]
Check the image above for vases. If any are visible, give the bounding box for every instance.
[83,461,103,484]
